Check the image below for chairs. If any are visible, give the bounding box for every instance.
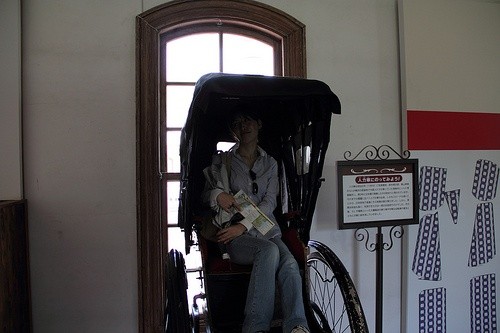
[199,228,308,276]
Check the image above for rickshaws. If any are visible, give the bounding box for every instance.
[163,73,368,333]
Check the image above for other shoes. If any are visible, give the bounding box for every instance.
[290,326,310,333]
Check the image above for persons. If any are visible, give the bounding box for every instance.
[201,109,310,333]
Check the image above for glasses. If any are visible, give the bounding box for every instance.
[249,169,259,196]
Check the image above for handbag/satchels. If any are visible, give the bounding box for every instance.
[198,209,225,244]
